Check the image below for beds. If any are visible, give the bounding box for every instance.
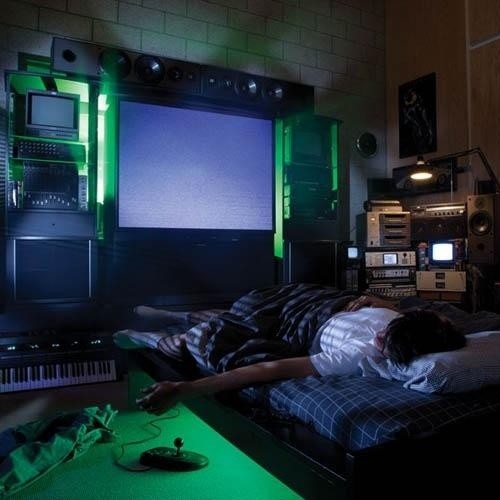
[116,303,498,499]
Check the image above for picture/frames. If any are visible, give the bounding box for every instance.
[397,73,438,160]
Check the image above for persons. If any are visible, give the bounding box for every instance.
[111,282,467,416]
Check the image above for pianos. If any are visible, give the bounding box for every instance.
[0,348,126,394]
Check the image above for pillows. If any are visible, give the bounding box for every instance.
[360,331,500,395]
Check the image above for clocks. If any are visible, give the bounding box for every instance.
[356,131,378,158]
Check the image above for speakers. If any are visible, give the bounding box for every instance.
[467,194,494,265]
[201,63,315,112]
[50,36,200,96]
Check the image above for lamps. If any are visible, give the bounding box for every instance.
[409,145,499,193]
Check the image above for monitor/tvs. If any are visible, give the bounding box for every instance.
[428,240,456,265]
[5,235,99,308]
[24,88,81,141]
